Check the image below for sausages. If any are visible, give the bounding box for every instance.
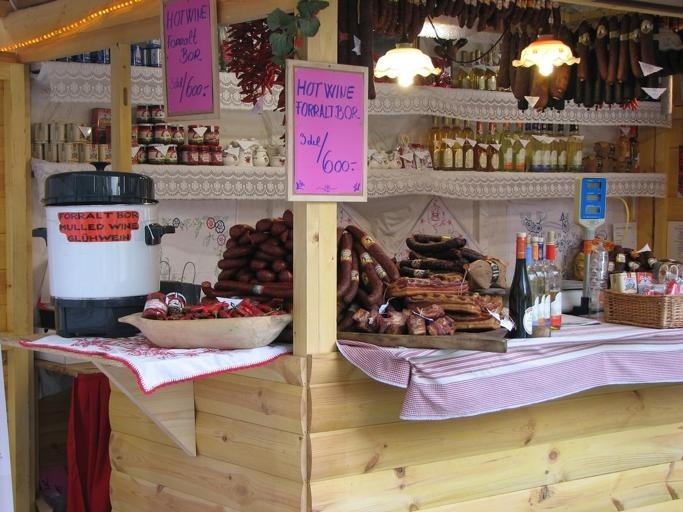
[200,206,487,333]
[369,0,682,112]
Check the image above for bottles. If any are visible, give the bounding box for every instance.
[104,101,224,170]
[507,230,562,339]
[137,290,189,321]
[583,239,610,314]
[426,48,585,172]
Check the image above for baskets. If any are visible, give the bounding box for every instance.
[603,287,682,329]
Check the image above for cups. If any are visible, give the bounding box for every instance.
[222,137,402,169]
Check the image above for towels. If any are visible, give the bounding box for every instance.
[16,335,293,395]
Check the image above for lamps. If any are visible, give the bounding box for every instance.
[373,0,442,88]
[512,0,581,75]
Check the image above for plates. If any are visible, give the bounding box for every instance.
[117,310,292,350]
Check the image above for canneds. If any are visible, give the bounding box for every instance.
[31,103,223,166]
[166,290,187,310]
[142,292,169,320]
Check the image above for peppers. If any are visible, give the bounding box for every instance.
[164,303,284,320]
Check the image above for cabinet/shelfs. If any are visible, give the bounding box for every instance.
[367,82,673,200]
[28,60,293,202]
